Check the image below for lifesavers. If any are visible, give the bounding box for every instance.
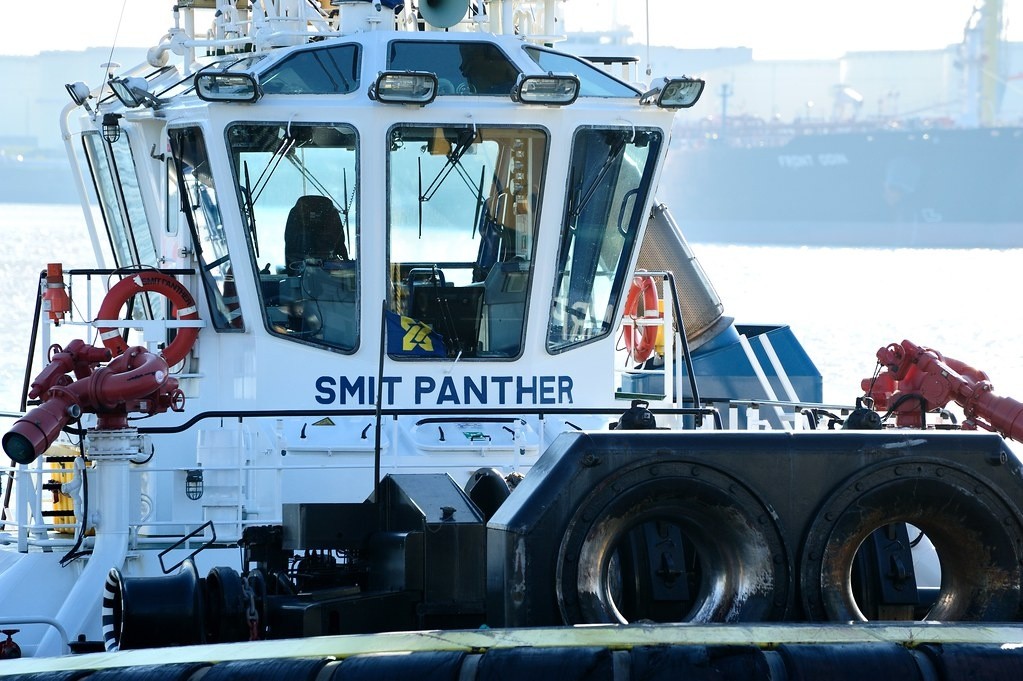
[97,272,199,367]
[623,274,659,362]
